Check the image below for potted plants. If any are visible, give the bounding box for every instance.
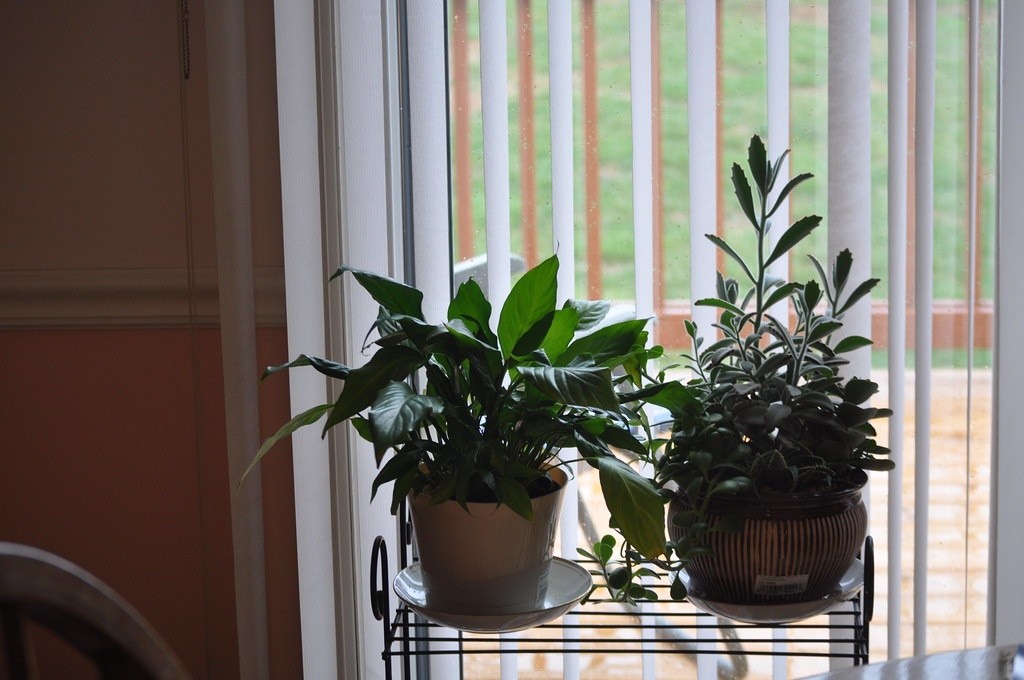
[238,238,669,635]
[575,134,895,624]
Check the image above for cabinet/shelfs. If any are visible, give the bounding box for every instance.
[180,0,286,328]
[1,0,193,329]
